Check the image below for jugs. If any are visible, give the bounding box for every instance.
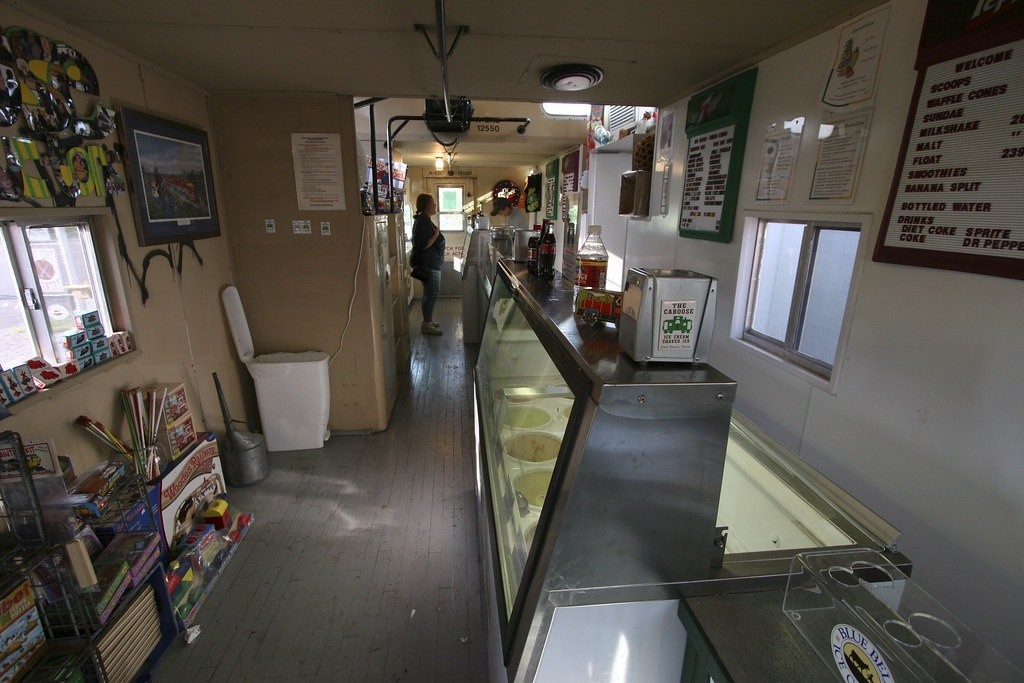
[212,371,268,488]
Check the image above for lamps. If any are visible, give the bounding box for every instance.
[436,157,443,170]
[539,63,604,92]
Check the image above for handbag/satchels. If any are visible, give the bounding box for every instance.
[410,264,432,283]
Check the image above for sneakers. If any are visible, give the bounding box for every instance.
[421,322,442,335]
[433,322,439,327]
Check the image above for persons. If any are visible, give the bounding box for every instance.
[409,194,445,334]
[74,154,88,183]
[24,32,58,64]
[0,169,24,202]
[188,169,208,206]
[490,198,526,230]
[154,167,167,209]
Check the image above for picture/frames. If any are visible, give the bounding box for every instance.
[114,107,221,247]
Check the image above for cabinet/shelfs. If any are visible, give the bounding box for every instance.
[0,432,179,683]
[390,254,405,378]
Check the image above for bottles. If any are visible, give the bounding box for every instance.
[538,223,557,279]
[572,224,609,314]
[526,225,542,274]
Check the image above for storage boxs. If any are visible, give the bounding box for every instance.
[94,433,229,573]
[140,382,198,461]
[618,169,650,218]
[175,512,255,631]
[0,579,46,683]
[0,308,133,406]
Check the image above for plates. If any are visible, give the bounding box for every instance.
[500,404,553,431]
[513,467,555,512]
[503,430,564,466]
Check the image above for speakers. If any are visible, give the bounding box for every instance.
[618,169,650,215]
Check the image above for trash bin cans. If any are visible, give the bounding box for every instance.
[221,285,330,452]
